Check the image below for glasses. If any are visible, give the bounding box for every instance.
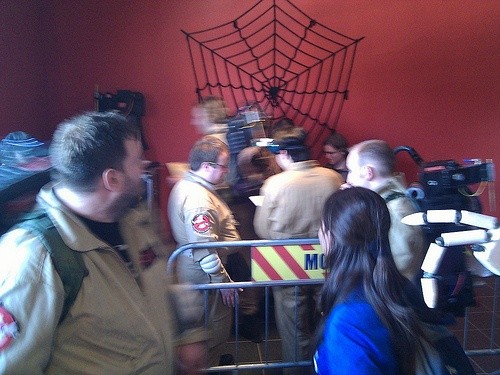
[201,162,229,169]
[322,150,339,154]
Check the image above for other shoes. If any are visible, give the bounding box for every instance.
[220,353,234,365]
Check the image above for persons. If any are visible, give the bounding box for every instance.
[167,98,437,375]
[0,110,215,375]
